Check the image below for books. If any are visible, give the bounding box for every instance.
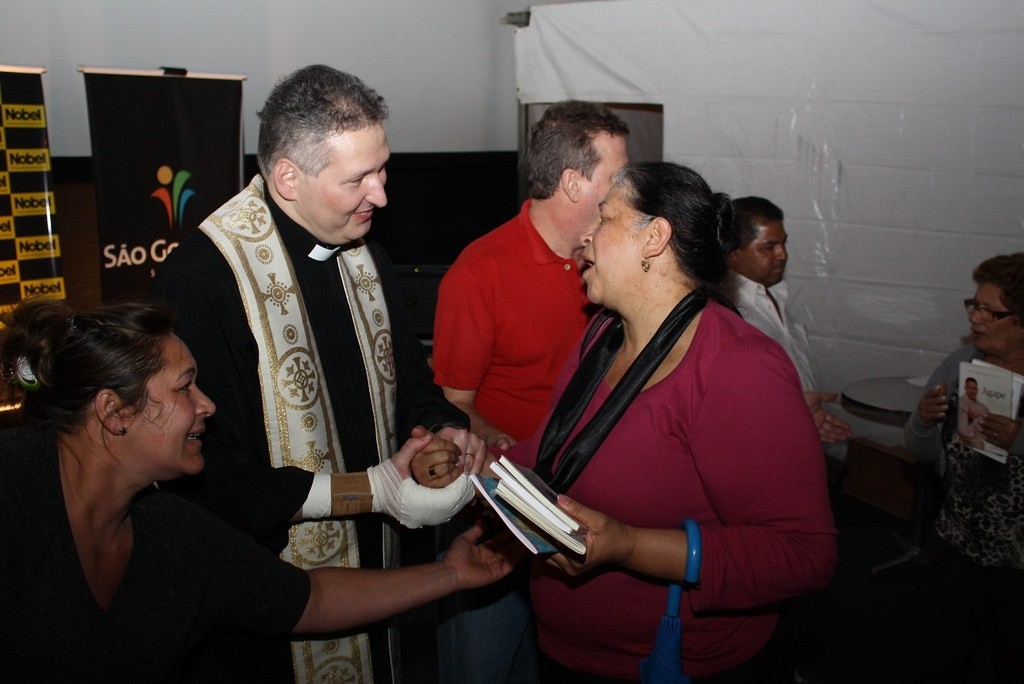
[956,359,1024,464]
[470,455,587,557]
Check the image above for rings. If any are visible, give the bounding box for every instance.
[994,433,997,437]
[429,466,438,480]
[466,453,475,458]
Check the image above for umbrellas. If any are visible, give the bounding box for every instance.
[639,518,701,684]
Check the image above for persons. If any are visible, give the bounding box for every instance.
[432,103,852,684]
[722,201,823,390]
[958,377,988,448]
[905,251,1024,683]
[0,300,526,683]
[144,65,485,683]
[412,161,839,683]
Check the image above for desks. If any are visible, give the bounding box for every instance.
[840,375,941,579]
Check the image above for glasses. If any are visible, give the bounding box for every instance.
[964,297,1018,322]
